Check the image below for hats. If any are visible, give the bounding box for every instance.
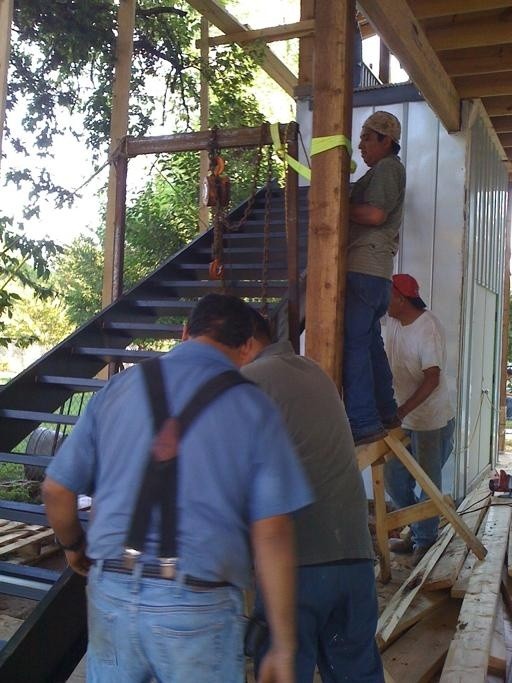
[391,273,426,308]
[363,111,401,145]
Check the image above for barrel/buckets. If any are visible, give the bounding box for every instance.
[25,426,64,481]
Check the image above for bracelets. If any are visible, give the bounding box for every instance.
[55,531,85,552]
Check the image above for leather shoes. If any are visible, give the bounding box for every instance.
[353,427,388,445]
[387,537,414,553]
[381,414,402,430]
[412,538,437,566]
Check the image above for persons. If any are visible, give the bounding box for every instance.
[238,307,390,682]
[39,291,318,681]
[334,109,405,448]
[504,368,512,421]
[382,271,458,569]
